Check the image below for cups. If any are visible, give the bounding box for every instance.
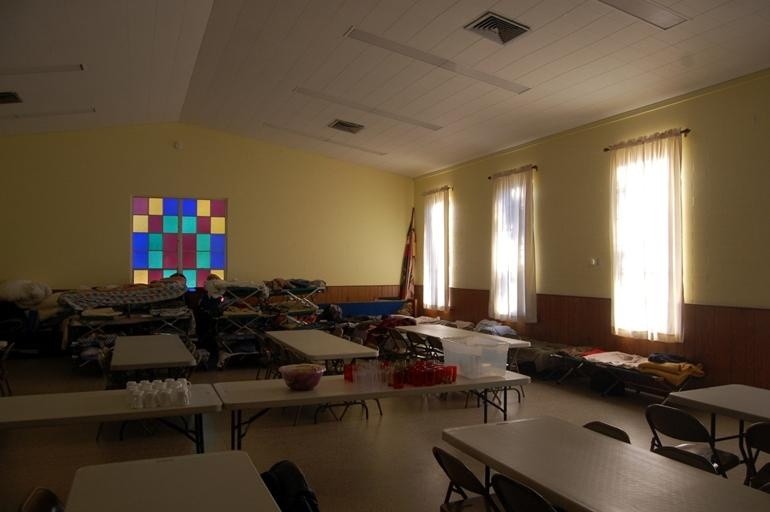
[127,377,194,408]
[340,359,458,392]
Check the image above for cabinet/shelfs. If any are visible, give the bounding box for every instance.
[62,312,195,361]
[216,280,326,370]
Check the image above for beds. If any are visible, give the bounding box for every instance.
[153,323,209,369]
[217,324,268,368]
[63,323,112,369]
[444,323,705,405]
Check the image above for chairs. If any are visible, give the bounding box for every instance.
[256,336,297,380]
[432,447,495,509]
[330,328,363,374]
[582,421,630,446]
[20,487,64,510]
[740,422,770,492]
[478,329,496,335]
[646,404,740,480]
[2,342,15,396]
[389,328,443,362]
[502,333,525,397]
[491,473,552,510]
[261,459,319,512]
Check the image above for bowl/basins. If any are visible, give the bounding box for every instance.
[279,363,328,391]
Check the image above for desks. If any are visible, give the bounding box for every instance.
[66,452,280,510]
[442,415,769,510]
[396,324,530,348]
[264,329,379,362]
[670,384,770,450]
[111,334,197,441]
[213,365,531,451]
[1,384,223,454]
